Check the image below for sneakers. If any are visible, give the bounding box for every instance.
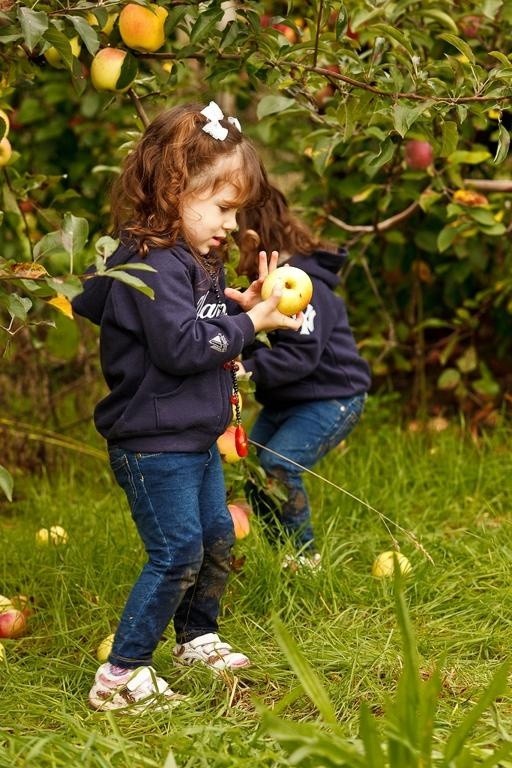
[87,665,194,718]
[171,634,254,678]
[279,554,324,578]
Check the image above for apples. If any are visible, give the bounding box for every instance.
[217,426,243,463]
[261,266,315,316]
[0,2,512,171]
[373,550,412,575]
[34,525,68,545]
[0,593,31,639]
[227,504,250,540]
[97,634,114,662]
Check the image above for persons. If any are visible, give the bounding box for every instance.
[212,179,372,580]
[67,101,304,718]
